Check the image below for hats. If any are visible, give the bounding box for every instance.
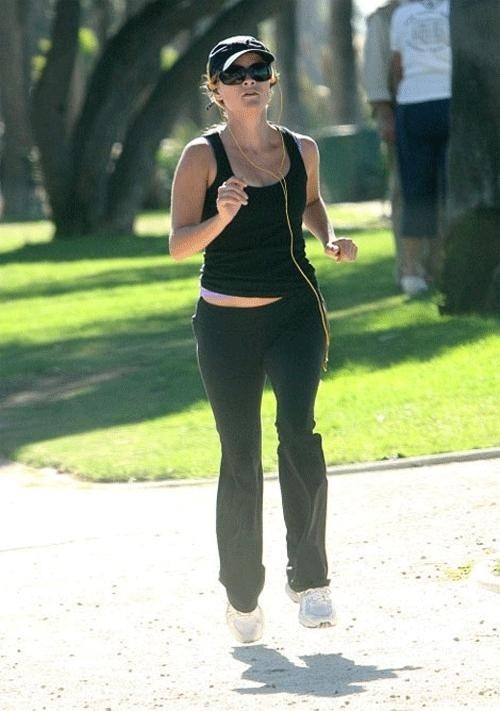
[206,34,276,76]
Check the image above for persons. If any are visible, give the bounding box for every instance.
[359,2,434,283]
[168,35,357,643]
[381,0,453,297]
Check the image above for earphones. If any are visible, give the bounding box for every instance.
[213,87,220,96]
[270,79,277,87]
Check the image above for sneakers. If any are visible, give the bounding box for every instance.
[284,580,337,629]
[226,597,265,645]
[400,273,428,297]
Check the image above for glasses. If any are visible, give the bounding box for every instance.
[218,60,273,87]
